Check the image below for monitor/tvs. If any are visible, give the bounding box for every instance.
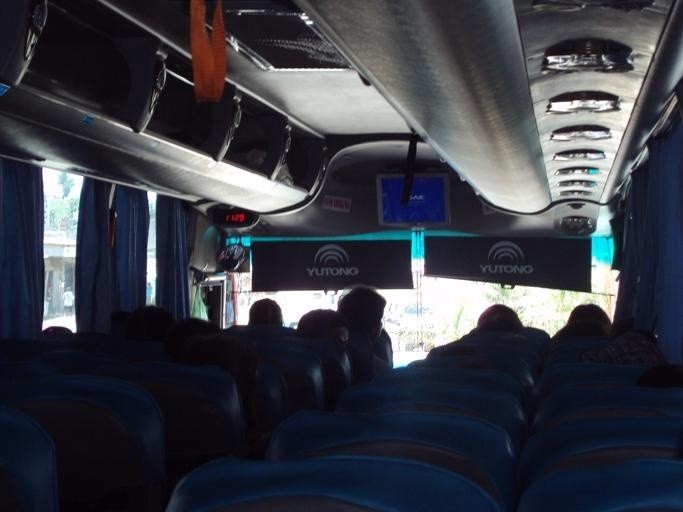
[376,173,451,229]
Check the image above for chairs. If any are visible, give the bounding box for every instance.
[1,325,682,512]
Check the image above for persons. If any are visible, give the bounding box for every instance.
[478,304,524,329]
[567,304,611,325]
[298,287,387,352]
[121,306,174,344]
[249,298,283,325]
